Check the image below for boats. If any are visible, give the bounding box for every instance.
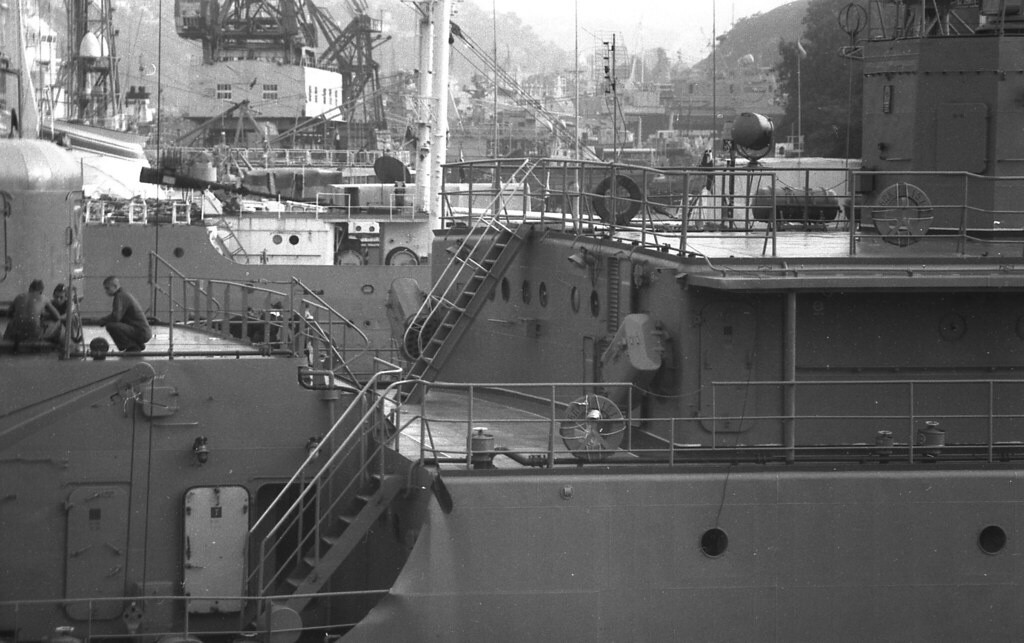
[1,0,1024,643]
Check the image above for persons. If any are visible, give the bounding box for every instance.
[3,278,81,352]
[96,276,153,353]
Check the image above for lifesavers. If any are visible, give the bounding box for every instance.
[592,174,642,226]
[559,393,627,461]
[871,183,935,247]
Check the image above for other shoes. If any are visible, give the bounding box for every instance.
[120,344,146,354]
